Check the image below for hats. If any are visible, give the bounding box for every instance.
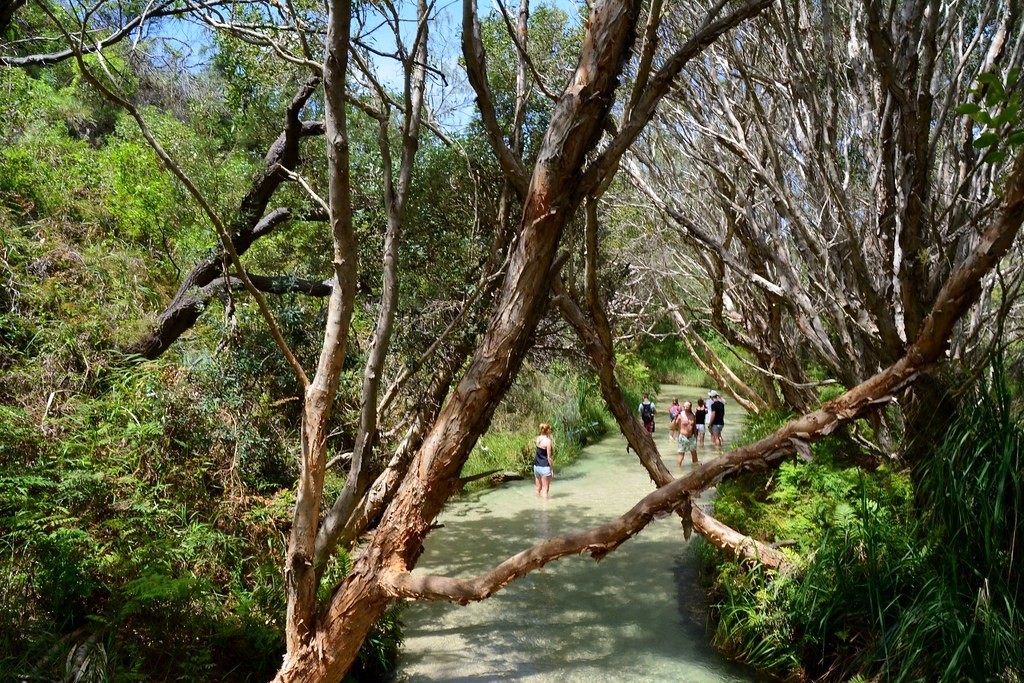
[708,390,718,396]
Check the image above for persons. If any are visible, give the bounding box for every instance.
[638,394,657,437]
[694,399,708,449]
[533,423,554,498]
[668,396,682,440]
[669,399,702,470]
[703,390,727,455]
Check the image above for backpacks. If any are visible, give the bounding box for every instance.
[641,402,654,423]
[670,406,680,420]
